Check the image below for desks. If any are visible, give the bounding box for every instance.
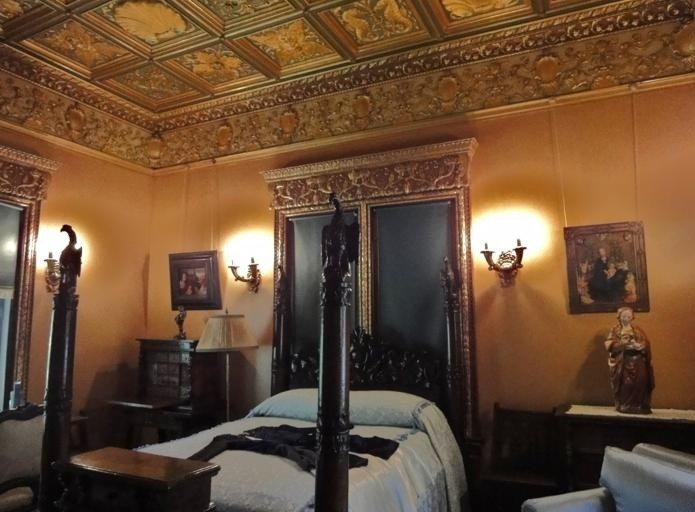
[105,392,188,446]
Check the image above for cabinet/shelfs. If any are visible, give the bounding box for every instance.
[132,334,223,447]
[552,400,695,494]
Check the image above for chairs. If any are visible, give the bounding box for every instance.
[518,438,695,511]
[479,398,569,511]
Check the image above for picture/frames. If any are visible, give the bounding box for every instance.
[166,249,222,313]
[561,222,649,313]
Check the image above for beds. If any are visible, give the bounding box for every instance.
[43,187,479,512]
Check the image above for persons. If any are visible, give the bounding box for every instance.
[179,272,207,295]
[590,247,626,302]
[604,307,655,414]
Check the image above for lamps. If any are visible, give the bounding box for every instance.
[195,302,260,423]
